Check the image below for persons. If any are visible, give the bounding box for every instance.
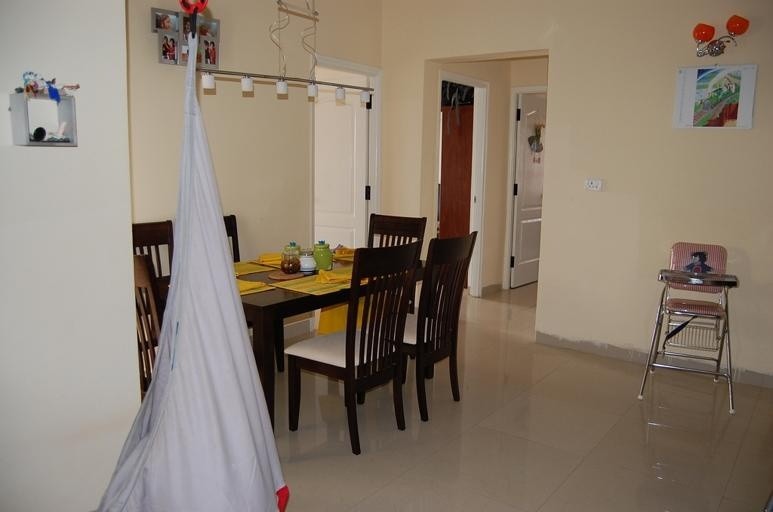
[204,40,210,65]
[209,42,215,65]
[160,15,171,30]
[183,21,193,41]
[161,36,168,57]
[164,38,174,60]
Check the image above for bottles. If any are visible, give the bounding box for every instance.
[280,240,332,275]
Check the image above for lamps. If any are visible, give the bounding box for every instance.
[691,14,750,58]
[198,0,374,104]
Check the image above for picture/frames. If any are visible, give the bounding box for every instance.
[149,8,219,69]
[672,65,758,130]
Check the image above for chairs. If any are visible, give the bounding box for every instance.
[132,215,175,303]
[133,255,162,404]
[355,230,479,422]
[284,239,424,456]
[638,242,738,415]
[223,216,286,372]
[367,216,428,361]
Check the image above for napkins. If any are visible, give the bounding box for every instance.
[333,252,354,262]
[238,281,266,292]
[316,270,353,284]
[259,251,281,265]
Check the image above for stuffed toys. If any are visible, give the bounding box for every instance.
[685,251,716,285]
